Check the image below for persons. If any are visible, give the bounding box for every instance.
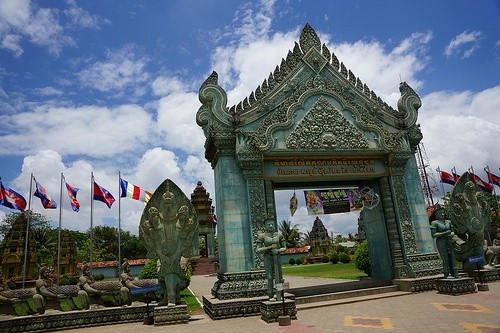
[0,274,35,315]
[256,215,287,301]
[121,261,161,304]
[33,267,80,314]
[78,265,123,310]
[483,239,500,266]
[431,204,462,278]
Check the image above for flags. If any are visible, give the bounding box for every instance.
[121,177,152,203]
[470,172,493,194]
[0,181,27,213]
[440,170,460,187]
[65,180,81,213]
[33,180,57,209]
[486,169,500,188]
[93,180,116,210]
